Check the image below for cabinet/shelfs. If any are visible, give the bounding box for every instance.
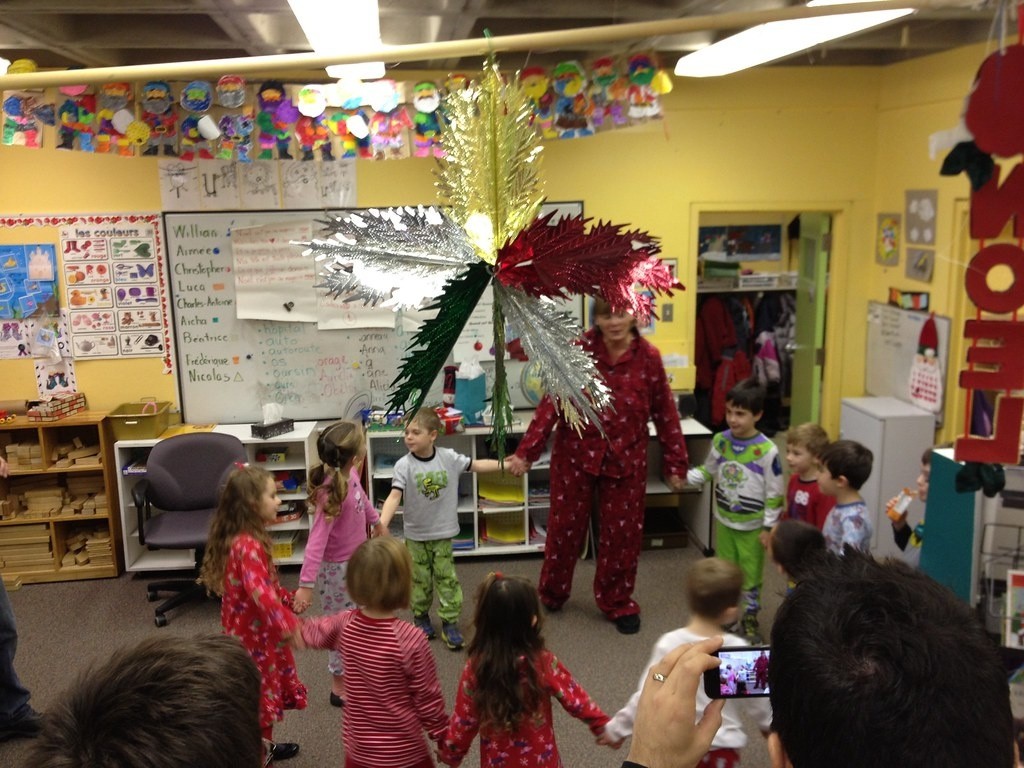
[0,410,126,585]
[113,422,324,582]
[365,411,557,565]
[919,447,986,611]
[839,397,936,572]
[588,417,716,558]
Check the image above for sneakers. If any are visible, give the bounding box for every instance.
[741,613,758,638]
[414,612,437,639]
[441,622,465,649]
[0,708,59,742]
[724,619,740,632]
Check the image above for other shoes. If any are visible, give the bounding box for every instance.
[329,690,347,707]
[616,613,640,633]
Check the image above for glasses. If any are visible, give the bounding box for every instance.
[260,736,277,768]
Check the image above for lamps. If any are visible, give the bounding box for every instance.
[674,0,916,79]
[285,0,386,80]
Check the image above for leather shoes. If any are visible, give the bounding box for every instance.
[274,743,299,760]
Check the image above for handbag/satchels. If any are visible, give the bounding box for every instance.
[763,380,784,408]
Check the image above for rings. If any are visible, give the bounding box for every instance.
[653,673,666,682]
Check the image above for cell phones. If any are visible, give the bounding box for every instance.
[703,645,771,699]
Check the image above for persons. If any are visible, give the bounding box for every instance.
[596,557,772,768]
[29,634,264,768]
[0,438,46,741]
[504,303,689,635]
[722,651,769,694]
[886,442,954,569]
[203,408,626,767]
[621,553,1015,768]
[670,380,874,646]
